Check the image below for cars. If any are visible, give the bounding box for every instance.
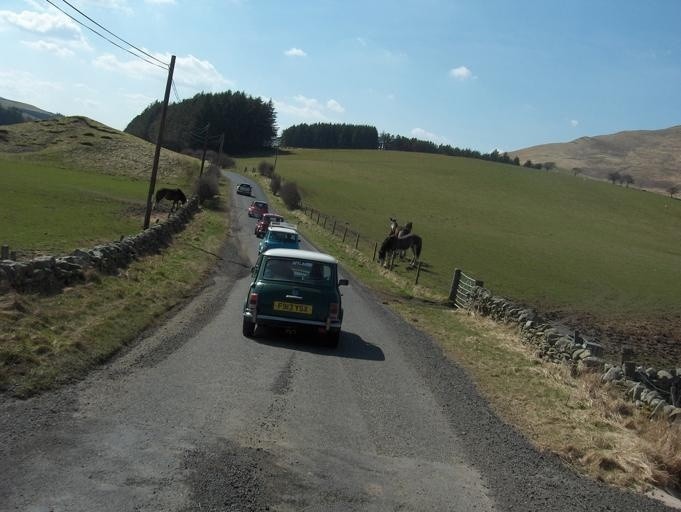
[248,200,301,253]
[235,183,253,197]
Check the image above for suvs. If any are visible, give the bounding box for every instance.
[242,248,349,348]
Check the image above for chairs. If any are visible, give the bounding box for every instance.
[270,262,325,284]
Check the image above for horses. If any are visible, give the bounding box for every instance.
[152,188,187,211]
[378,234,422,268]
